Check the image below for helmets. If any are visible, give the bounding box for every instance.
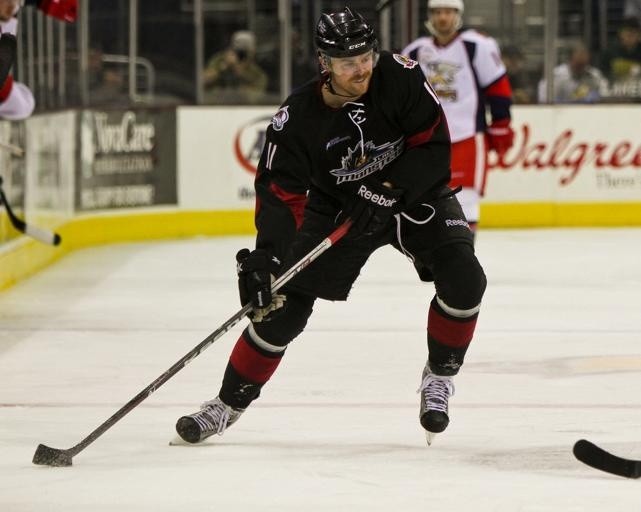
[424,0,464,39]
[314,5,380,83]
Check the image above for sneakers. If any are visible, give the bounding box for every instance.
[417,360,455,432]
[176,395,246,444]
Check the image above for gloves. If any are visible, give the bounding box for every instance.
[341,180,403,234]
[236,248,290,324]
[487,126,514,154]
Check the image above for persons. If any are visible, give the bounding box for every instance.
[402,0,513,239]
[65,49,127,104]
[203,22,316,105]
[176,8,488,445]
[504,7,641,104]
[0,0,78,122]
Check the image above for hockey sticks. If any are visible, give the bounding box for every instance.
[1,189,61,245]
[573,438,641,479]
[32,218,355,466]
